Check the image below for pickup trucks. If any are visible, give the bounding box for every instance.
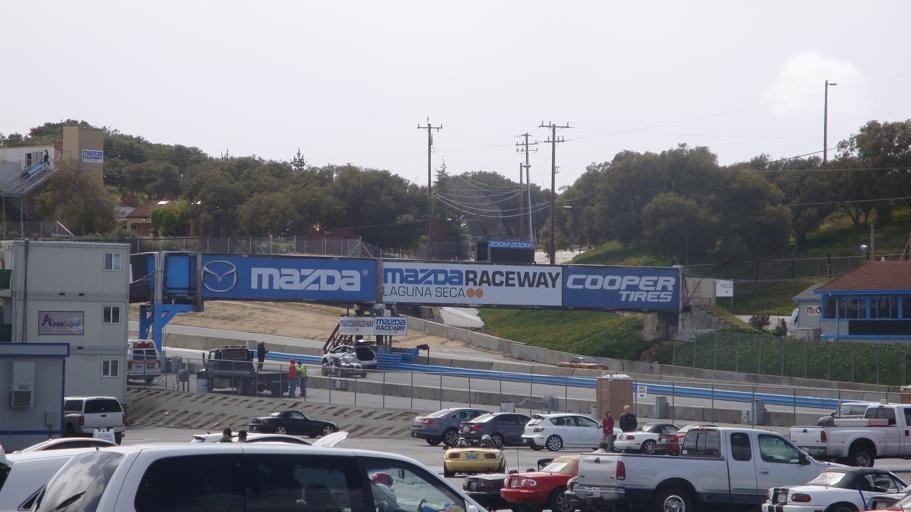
[572,426,850,512]
[789,403,911,468]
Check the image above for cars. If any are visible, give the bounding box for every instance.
[669,423,717,456]
[653,424,717,456]
[0,431,493,511]
[322,357,368,379]
[557,356,610,372]
[410,408,491,446]
[860,484,911,512]
[458,412,532,448]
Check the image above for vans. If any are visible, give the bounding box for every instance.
[127,339,162,387]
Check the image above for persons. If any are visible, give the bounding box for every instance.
[221,427,232,443]
[782,319,787,337]
[288,360,298,397]
[237,430,247,443]
[256,342,268,372]
[598,411,614,452]
[297,362,308,396]
[619,405,638,433]
[44,150,49,172]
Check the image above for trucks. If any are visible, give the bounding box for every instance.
[194,344,299,394]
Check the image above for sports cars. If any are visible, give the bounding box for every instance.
[462,458,554,511]
[249,409,337,439]
[761,467,909,512]
[613,423,682,455]
[499,456,580,512]
[442,432,508,477]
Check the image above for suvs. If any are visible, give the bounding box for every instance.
[63,396,128,445]
[521,413,624,452]
[321,338,379,368]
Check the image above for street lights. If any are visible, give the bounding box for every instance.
[860,242,870,260]
[546,204,573,255]
[821,75,839,163]
